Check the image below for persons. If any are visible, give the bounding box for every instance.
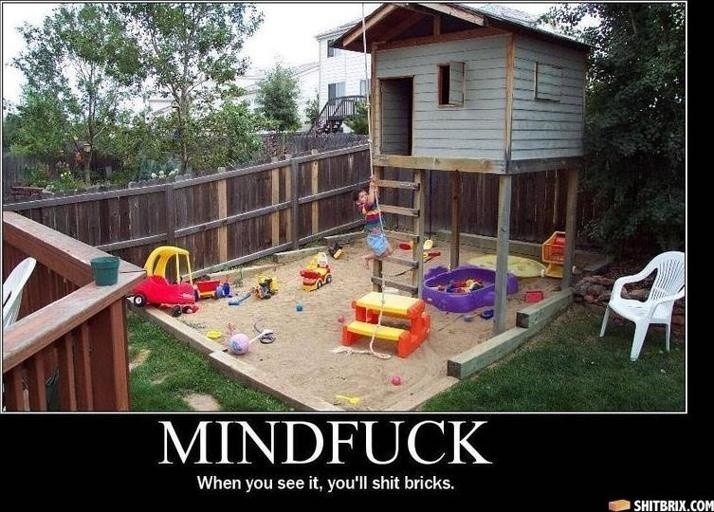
[130,239,518,385]
[130,239,518,387]
[351,176,393,270]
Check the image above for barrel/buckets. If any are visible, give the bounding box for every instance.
[90,258,119,285]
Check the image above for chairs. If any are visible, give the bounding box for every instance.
[598,250,686,361]
